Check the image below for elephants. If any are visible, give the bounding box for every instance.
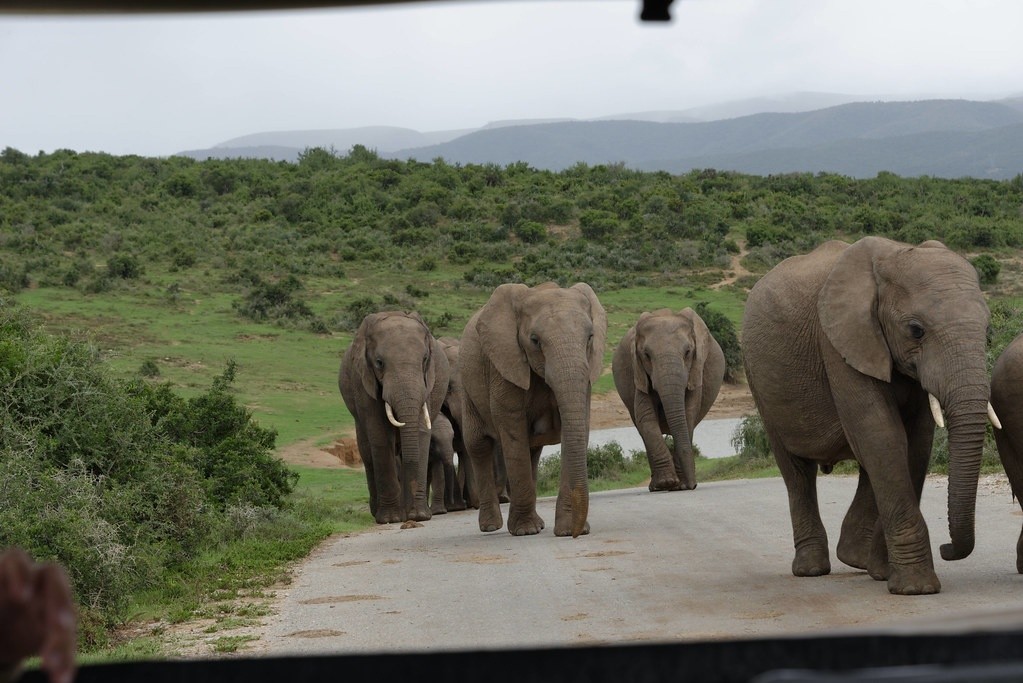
[741,236,1023,595]
[338,282,606,539]
[613,306,725,491]
